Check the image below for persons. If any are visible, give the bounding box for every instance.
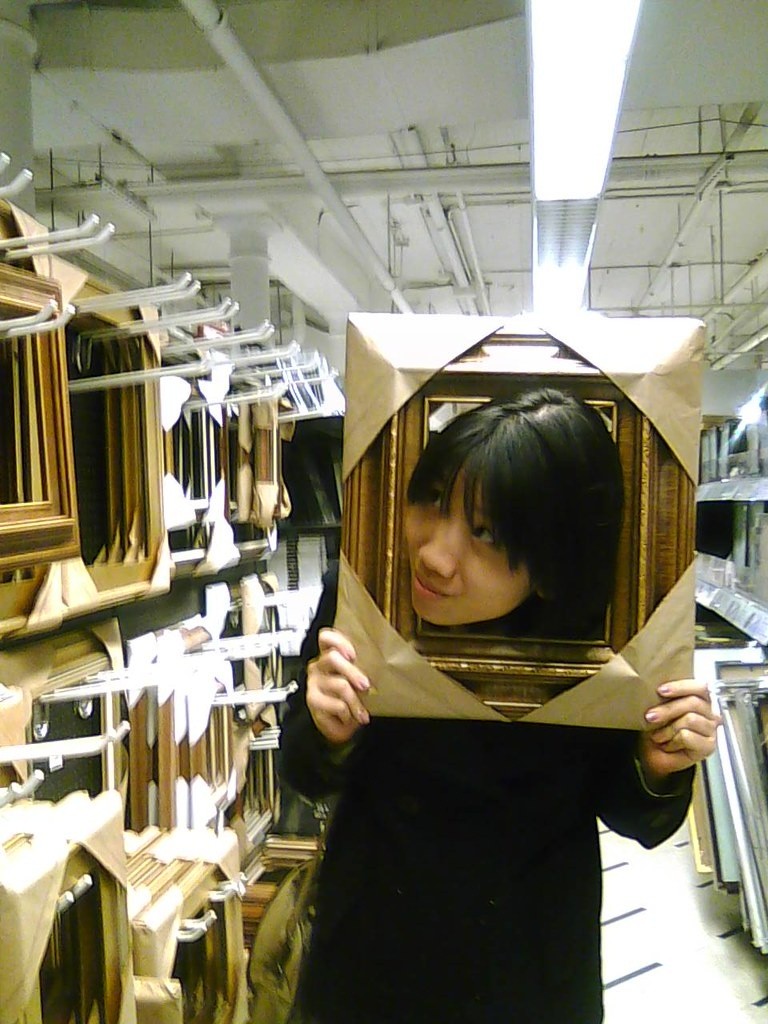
[271,387,724,1024]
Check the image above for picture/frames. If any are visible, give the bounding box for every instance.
[0,204,768,1024]
